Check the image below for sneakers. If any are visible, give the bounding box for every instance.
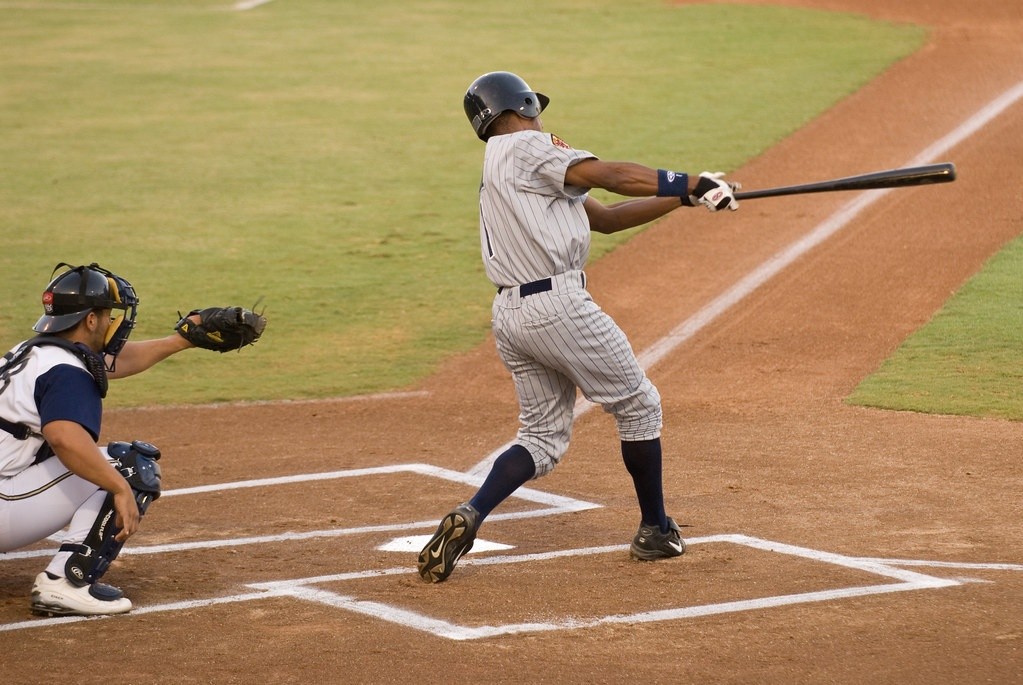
[417,503,481,583]
[28,572,133,615]
[630,516,687,560]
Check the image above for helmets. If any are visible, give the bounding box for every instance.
[462,71,550,142]
[33,265,113,333]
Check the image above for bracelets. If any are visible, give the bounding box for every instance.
[657,168,688,198]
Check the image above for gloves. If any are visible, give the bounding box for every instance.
[692,176,742,212]
[680,171,726,208]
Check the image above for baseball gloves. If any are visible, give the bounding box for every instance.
[173,295,270,353]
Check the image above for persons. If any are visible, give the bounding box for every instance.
[416,70,742,584]
[0,260,267,616]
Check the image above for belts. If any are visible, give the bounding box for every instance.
[498,272,585,299]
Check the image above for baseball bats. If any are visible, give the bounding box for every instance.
[732,162,957,200]
